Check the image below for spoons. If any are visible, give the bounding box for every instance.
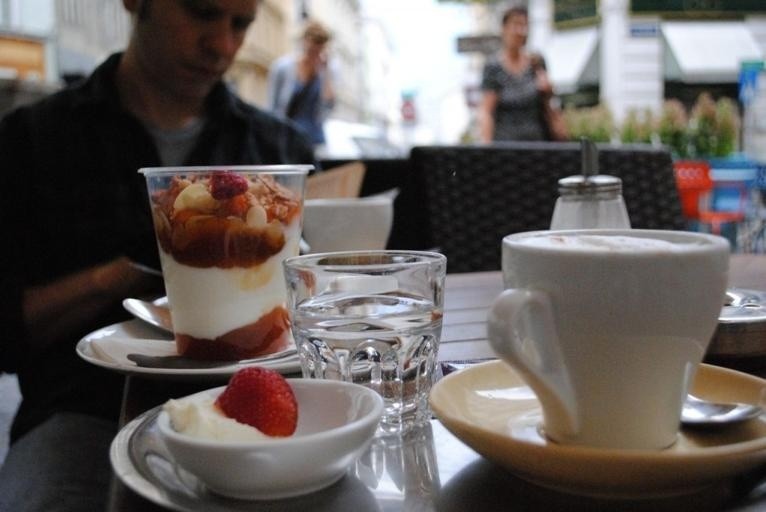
[680,394,764,435]
[121,295,174,339]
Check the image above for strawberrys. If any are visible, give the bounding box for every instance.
[213,366,298,439]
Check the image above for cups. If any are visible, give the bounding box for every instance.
[296,196,393,253]
[489,227,735,454]
[132,162,315,363]
[283,251,448,439]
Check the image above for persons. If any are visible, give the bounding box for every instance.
[479,8,567,145]
[0,0,313,512]
[267,24,337,145]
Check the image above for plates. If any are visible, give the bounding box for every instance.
[70,319,309,379]
[157,377,386,499]
[426,357,766,503]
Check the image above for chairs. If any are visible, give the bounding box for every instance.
[671,156,746,236]
[385,147,692,272]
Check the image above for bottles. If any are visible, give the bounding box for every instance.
[549,173,631,227]
